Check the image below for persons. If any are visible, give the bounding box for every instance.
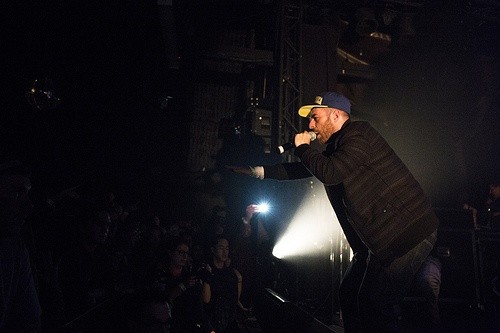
[0,168,281,333]
[480,180,500,216]
[227,92,440,333]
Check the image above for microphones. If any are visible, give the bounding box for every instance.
[277,132,316,155]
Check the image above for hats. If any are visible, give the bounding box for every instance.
[298,92,351,117]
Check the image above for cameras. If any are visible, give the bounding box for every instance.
[194,264,215,283]
